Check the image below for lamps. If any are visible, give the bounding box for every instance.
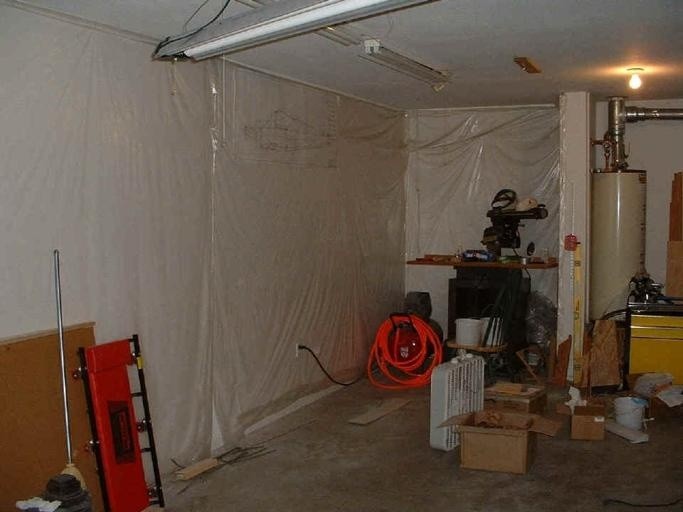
[149,1,433,64]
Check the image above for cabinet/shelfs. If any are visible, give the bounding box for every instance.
[628,313,683,390]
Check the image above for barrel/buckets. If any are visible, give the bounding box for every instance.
[455,318,483,345]
[614,397,648,430]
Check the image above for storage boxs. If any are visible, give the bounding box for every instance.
[434,381,616,477]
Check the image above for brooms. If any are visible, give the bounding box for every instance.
[54,248,92,512]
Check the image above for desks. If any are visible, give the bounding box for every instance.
[406,257,556,381]
